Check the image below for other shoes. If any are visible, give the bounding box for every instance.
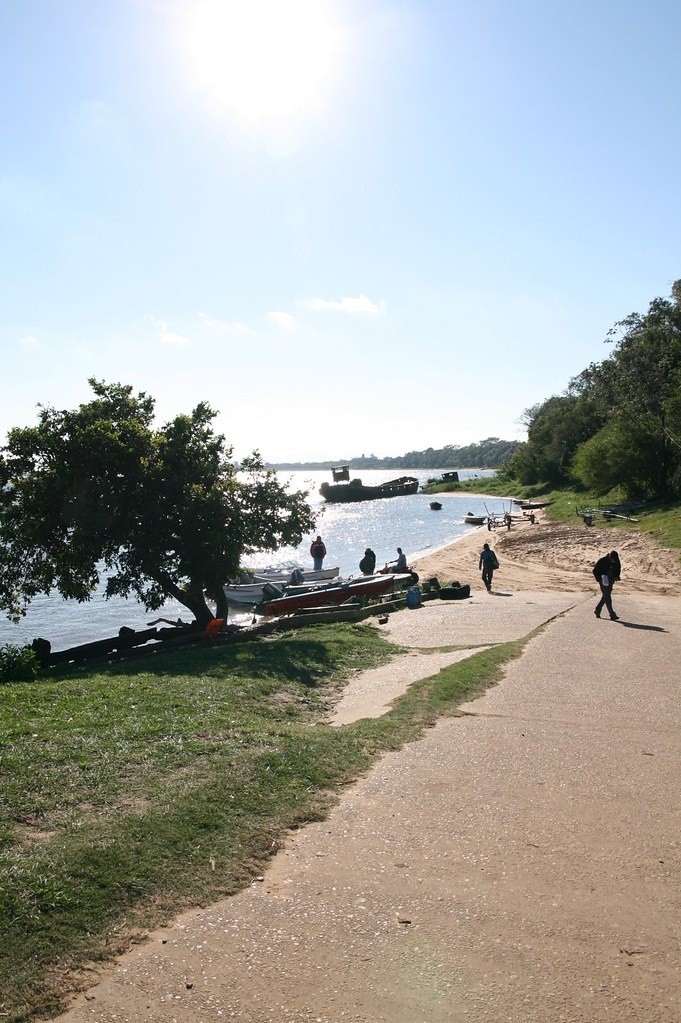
[594,609,601,618]
[487,586,491,591]
[609,612,620,620]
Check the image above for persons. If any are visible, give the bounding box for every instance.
[592,551,621,620]
[390,548,406,572]
[310,536,326,571]
[359,548,376,575]
[479,544,499,591]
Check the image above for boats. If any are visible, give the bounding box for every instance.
[318,475,419,503]
[429,501,442,510]
[202,559,412,617]
[330,465,350,483]
[520,500,556,510]
[461,515,487,525]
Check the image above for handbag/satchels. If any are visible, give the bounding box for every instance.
[491,551,499,569]
